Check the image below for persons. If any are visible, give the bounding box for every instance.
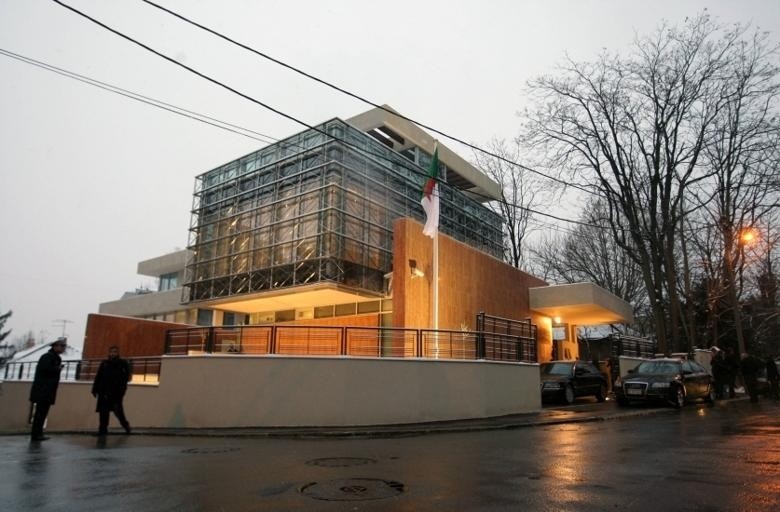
[90,343,133,434]
[709,345,779,405]
[26,336,69,441]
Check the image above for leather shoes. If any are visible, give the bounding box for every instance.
[125,422,130,434]
[97,437,106,448]
[32,435,49,440]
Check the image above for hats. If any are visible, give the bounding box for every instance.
[49,337,66,347]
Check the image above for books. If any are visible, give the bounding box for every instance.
[419,145,440,245]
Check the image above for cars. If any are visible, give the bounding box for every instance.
[613,354,718,410]
[539,360,608,407]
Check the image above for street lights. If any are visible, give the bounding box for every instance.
[725,226,754,393]
